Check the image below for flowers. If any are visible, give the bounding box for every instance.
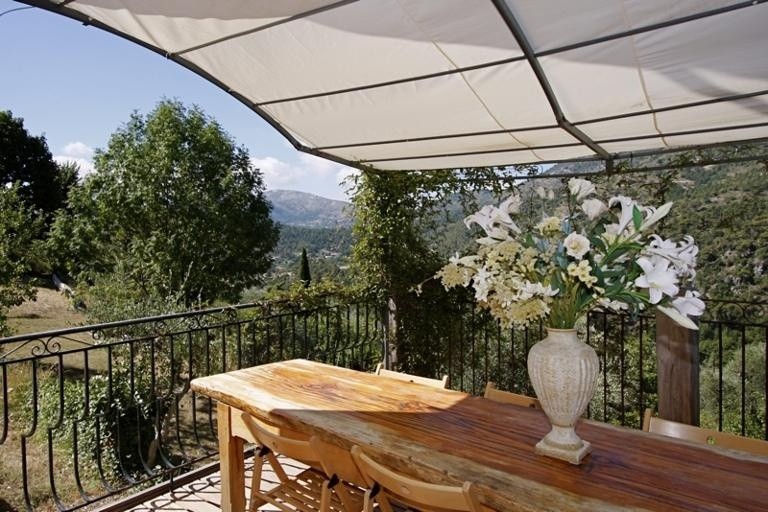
[406,179,713,336]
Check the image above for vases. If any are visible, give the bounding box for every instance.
[526,326,602,467]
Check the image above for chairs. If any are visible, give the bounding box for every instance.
[349,442,483,511]
[373,362,450,389]
[640,406,768,462]
[481,380,542,408]
[238,410,366,511]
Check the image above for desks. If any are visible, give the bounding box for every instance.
[189,356,768,511]
